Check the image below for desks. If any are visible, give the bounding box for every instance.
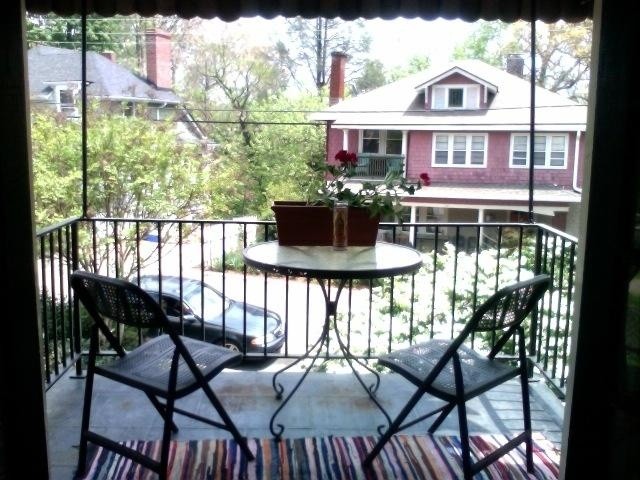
[243,239,427,437]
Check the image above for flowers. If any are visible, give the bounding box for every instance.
[304,147,432,232]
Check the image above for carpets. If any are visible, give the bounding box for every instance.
[73,431,562,480]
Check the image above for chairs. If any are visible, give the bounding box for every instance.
[65,268,254,480]
[364,275,556,480]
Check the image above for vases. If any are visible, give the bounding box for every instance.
[272,197,380,248]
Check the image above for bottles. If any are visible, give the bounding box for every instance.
[332,201,348,251]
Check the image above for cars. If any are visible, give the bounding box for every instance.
[128,274,284,369]
